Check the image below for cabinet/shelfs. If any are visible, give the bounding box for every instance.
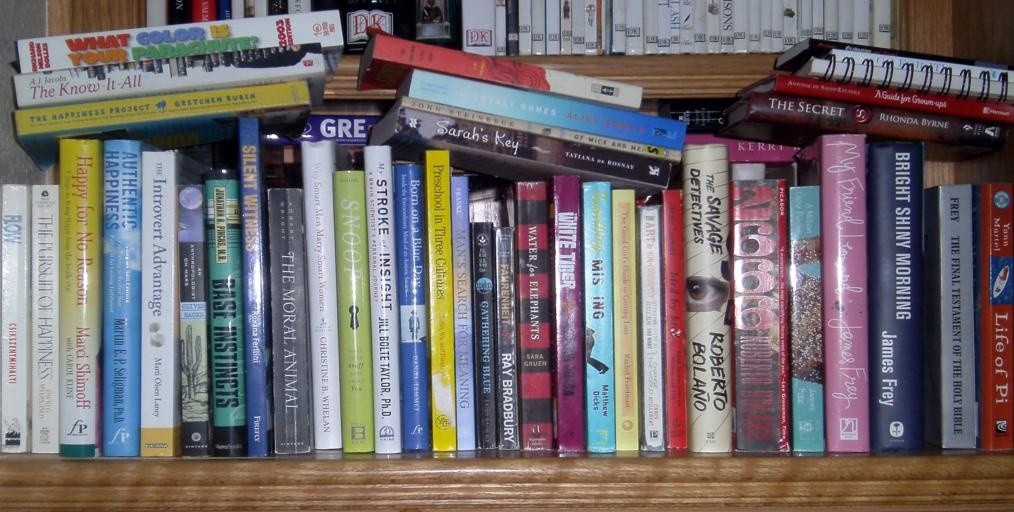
[0,1,1013,512]
